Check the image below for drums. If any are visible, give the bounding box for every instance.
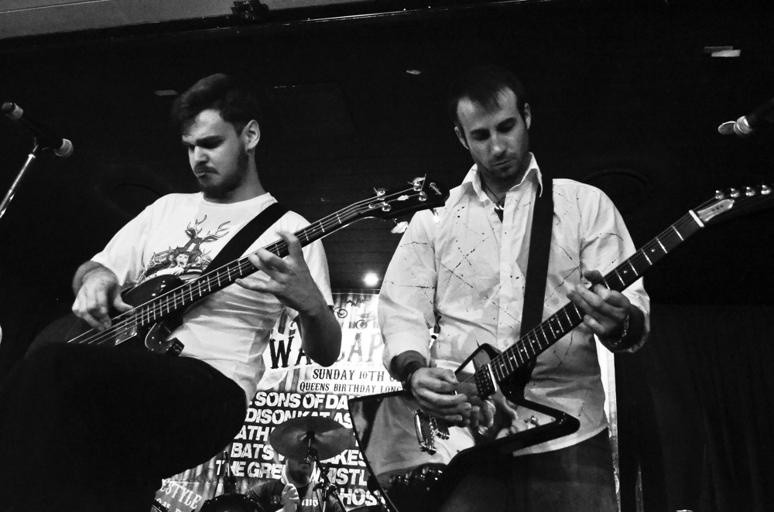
[200,494,265,512]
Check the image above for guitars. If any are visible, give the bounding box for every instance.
[348,185,772,508]
[20,176,448,416]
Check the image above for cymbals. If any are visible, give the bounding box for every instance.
[270,416,355,460]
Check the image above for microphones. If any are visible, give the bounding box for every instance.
[732,97,774,139]
[2,99,73,159]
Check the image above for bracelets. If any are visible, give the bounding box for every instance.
[606,315,633,350]
[402,360,423,391]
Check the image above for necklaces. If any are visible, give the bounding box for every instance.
[482,178,506,223]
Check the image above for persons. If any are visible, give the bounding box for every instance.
[380,66,650,512]
[70,70,342,512]
[245,457,346,512]
[0,248,247,512]
